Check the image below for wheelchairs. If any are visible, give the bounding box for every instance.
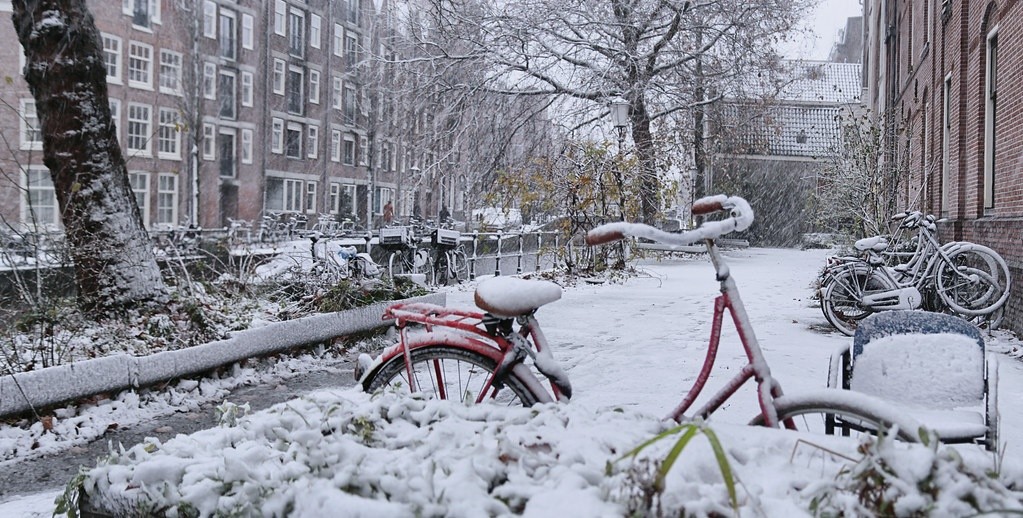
[826,309,1005,448]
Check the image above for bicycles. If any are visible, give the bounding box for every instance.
[812,213,1012,332]
[353,194,934,447]
[1,228,68,267]
[303,226,471,298]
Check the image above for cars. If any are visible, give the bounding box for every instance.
[479,212,520,231]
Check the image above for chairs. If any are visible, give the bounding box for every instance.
[825,311,999,452]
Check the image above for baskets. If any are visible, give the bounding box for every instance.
[431,229,460,249]
[380,228,412,250]
[934,219,962,246]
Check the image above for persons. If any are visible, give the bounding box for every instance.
[383,201,394,224]
[440,205,450,222]
[476,214,483,221]
